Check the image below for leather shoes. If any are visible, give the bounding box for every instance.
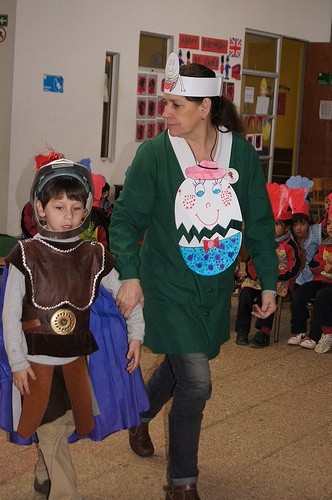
[129,422,154,457]
[164,485,201,500]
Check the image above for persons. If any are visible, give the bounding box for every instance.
[233,177,332,353]
[0,158,144,500]
[109,64,279,500]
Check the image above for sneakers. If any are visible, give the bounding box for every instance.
[315,333,332,354]
[300,338,317,348]
[287,333,307,345]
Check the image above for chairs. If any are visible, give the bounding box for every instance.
[309,177,332,220]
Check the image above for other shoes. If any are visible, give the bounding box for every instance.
[249,330,271,348]
[236,331,250,346]
[34,478,52,495]
